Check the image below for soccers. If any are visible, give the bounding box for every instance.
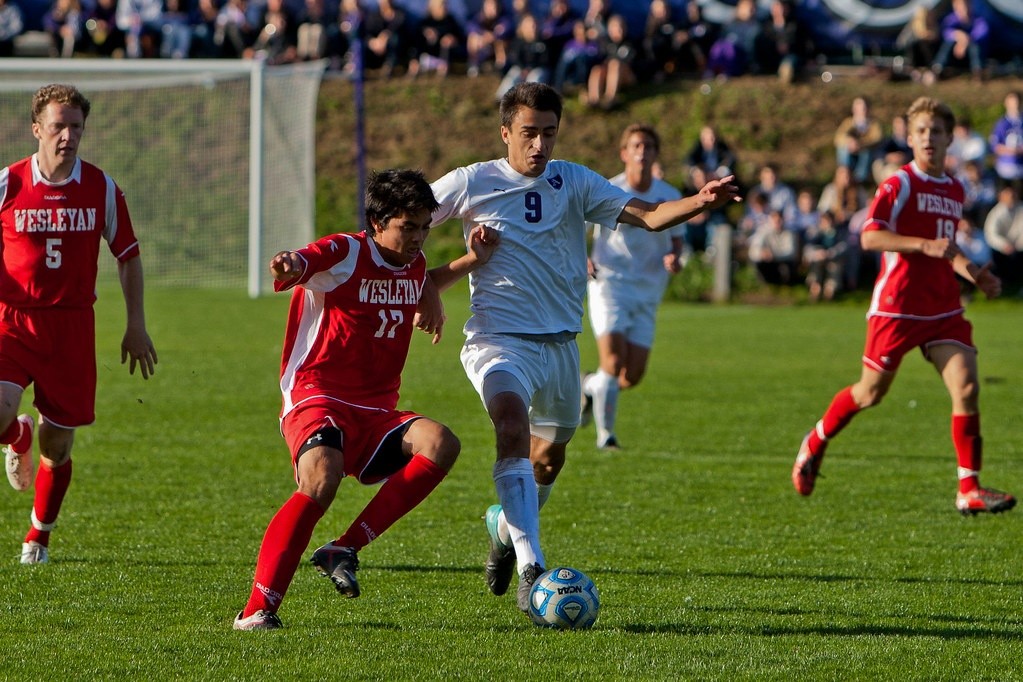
[528,565,601,630]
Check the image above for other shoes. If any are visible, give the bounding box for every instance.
[580,371,592,427]
[598,435,621,451]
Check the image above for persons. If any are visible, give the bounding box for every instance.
[0,84,159,564]
[791,97,1017,518]
[894,0,989,88]
[410,81,743,614]
[649,90,1023,307]
[0,0,818,115]
[577,123,686,449]
[233,168,501,630]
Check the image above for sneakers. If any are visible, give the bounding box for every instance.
[956,487,1017,517]
[13,541,47,566]
[233,607,284,632]
[2,414,35,491]
[791,435,828,496]
[481,504,516,596]
[309,539,360,598]
[517,561,546,612]
